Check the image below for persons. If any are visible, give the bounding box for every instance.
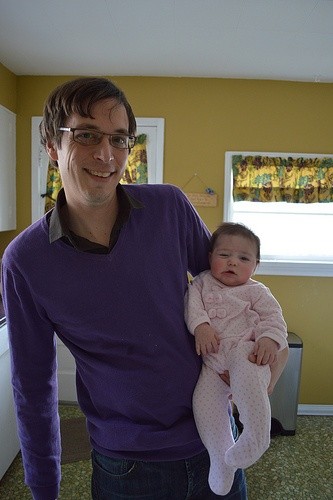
[185,222,288,496]
[1,77,249,500]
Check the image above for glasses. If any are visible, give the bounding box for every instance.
[58,126,137,149]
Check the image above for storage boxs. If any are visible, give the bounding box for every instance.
[233,331,303,435]
